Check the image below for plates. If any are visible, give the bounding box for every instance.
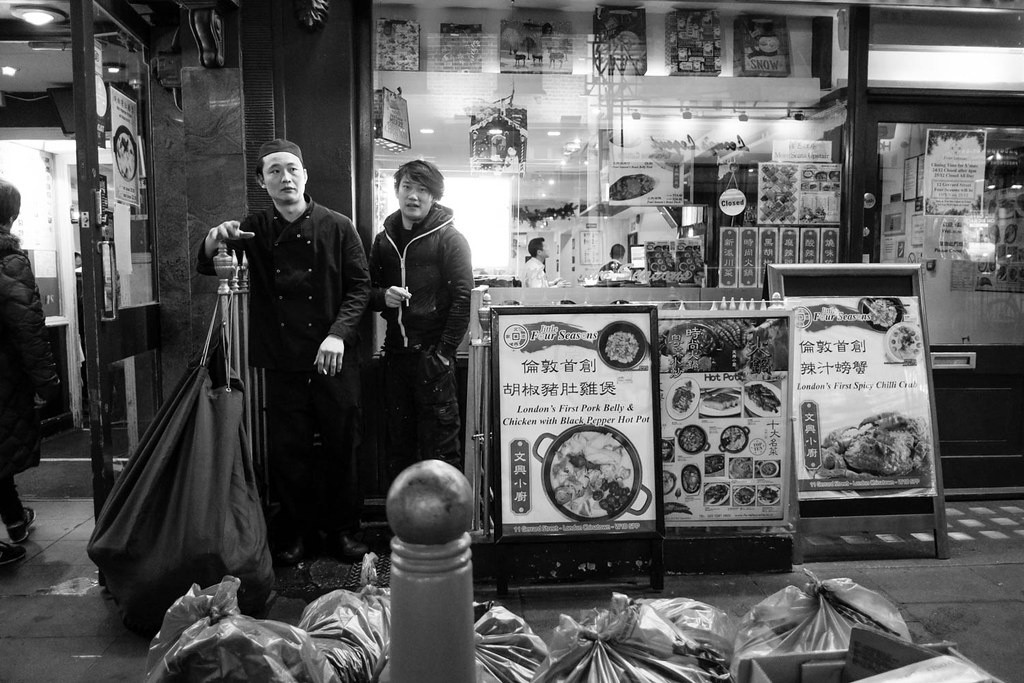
[662,378,780,506]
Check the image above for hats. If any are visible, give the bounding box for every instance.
[257,140,303,164]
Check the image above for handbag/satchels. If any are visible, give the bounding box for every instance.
[86,289,275,638]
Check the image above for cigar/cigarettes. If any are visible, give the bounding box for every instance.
[323,368,327,374]
[406,286,409,306]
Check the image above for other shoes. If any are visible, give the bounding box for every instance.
[275,539,307,564]
[333,533,369,563]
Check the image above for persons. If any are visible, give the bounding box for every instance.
[522,237,571,288]
[196,138,374,563]
[367,160,476,485]
[599,243,626,279]
[0,178,62,565]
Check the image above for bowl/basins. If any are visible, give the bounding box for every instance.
[594,321,651,371]
[857,298,905,333]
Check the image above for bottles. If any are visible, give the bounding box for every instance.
[388,460,476,682]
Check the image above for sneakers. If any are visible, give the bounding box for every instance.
[0,540,27,565]
[7,506,37,544]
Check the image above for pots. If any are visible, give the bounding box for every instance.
[718,426,750,454]
[532,423,652,524]
[674,426,711,455]
